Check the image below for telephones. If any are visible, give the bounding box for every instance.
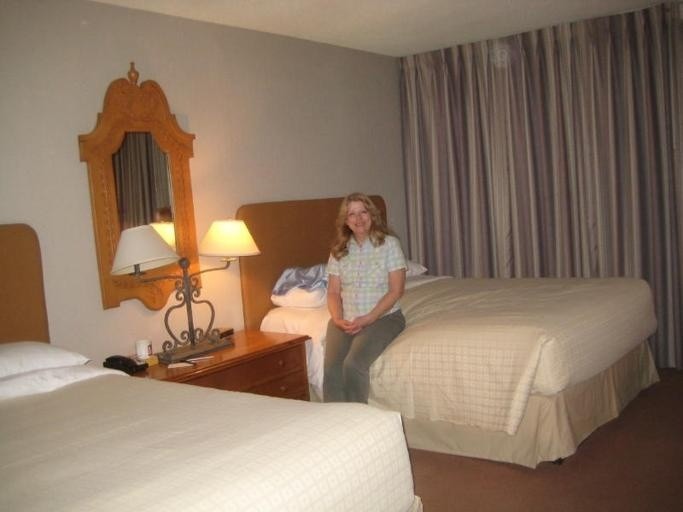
[105,356,148,375]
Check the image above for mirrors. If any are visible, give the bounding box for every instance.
[79,62,202,310]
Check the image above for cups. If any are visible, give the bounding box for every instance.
[136,339,153,360]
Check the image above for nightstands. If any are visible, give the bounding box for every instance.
[135,329,313,403]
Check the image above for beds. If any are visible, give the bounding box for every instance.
[236,197,657,467]
[1,221,422,512]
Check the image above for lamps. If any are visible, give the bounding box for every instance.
[113,218,260,362]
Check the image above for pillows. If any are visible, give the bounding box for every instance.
[1,341,90,376]
[272,260,428,307]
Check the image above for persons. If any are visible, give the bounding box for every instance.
[322,192,408,405]
[153,206,171,223]
